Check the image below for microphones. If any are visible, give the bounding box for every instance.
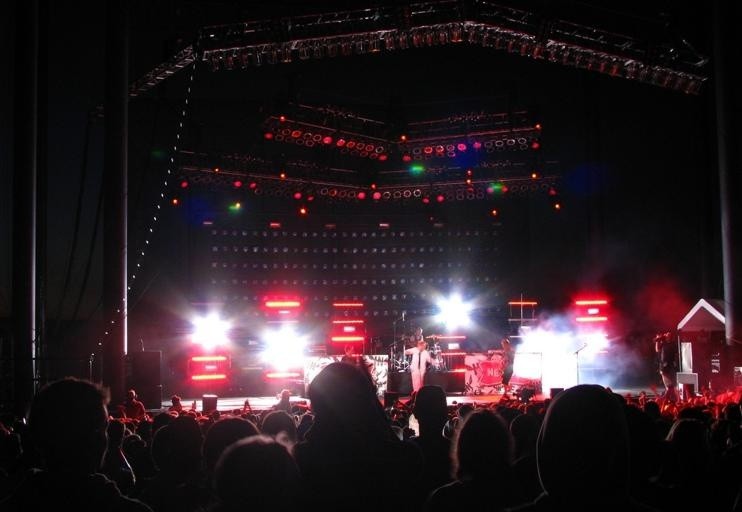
[401,312,406,321]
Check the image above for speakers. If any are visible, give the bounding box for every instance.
[126,351,161,409]
[387,370,413,396]
[677,331,734,395]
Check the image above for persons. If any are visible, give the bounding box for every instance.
[501,339,515,396]
[406,329,433,390]
[0,361,742,512]
[342,344,356,363]
[655,331,678,401]
